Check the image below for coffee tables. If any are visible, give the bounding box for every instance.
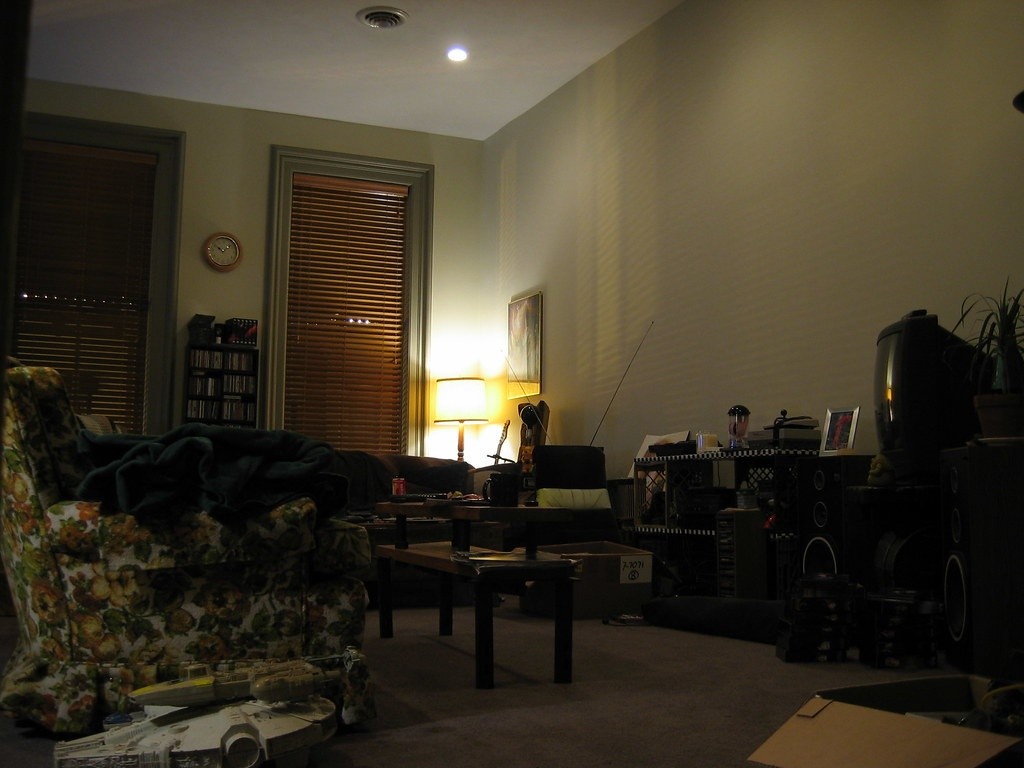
[374,496,577,689]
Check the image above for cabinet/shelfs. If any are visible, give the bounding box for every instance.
[183,347,263,427]
[632,446,820,597]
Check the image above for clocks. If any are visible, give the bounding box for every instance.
[203,230,244,274]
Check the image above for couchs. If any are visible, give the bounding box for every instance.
[0,353,472,737]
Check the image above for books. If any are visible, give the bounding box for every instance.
[187,351,255,422]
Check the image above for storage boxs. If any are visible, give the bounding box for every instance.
[746,428,821,450]
[227,318,259,345]
[517,539,662,621]
[747,673,1024,768]
[187,314,215,344]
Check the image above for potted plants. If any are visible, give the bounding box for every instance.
[943,277,1024,437]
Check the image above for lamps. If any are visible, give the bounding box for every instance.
[518,400,542,474]
[727,404,752,448]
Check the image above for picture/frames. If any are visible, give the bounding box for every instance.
[816,405,862,453]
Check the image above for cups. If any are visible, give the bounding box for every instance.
[695,429,719,453]
[483,472,521,507]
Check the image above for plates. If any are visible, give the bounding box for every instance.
[467,499,485,505]
[523,502,538,507]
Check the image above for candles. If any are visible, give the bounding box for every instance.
[695,430,718,452]
[391,473,408,496]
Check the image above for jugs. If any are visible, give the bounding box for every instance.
[727,405,751,448]
